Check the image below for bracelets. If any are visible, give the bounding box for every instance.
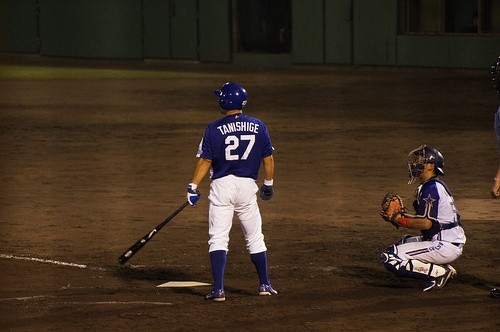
[494,177,500,181]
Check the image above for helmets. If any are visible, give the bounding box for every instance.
[213,81,247,109]
[414,145,446,177]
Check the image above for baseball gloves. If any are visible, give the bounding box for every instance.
[380,192,406,228]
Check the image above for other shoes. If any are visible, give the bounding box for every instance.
[259,283,278,295]
[206,288,225,301]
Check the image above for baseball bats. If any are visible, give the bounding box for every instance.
[117,191,201,265]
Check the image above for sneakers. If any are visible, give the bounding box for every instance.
[422,263,457,292]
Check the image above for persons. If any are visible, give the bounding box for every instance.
[380,145,466,292]
[270,0,289,53]
[186,83,278,301]
[488,55,500,297]
[463,12,482,33]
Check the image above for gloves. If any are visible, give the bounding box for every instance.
[260,178,274,199]
[186,182,200,205]
